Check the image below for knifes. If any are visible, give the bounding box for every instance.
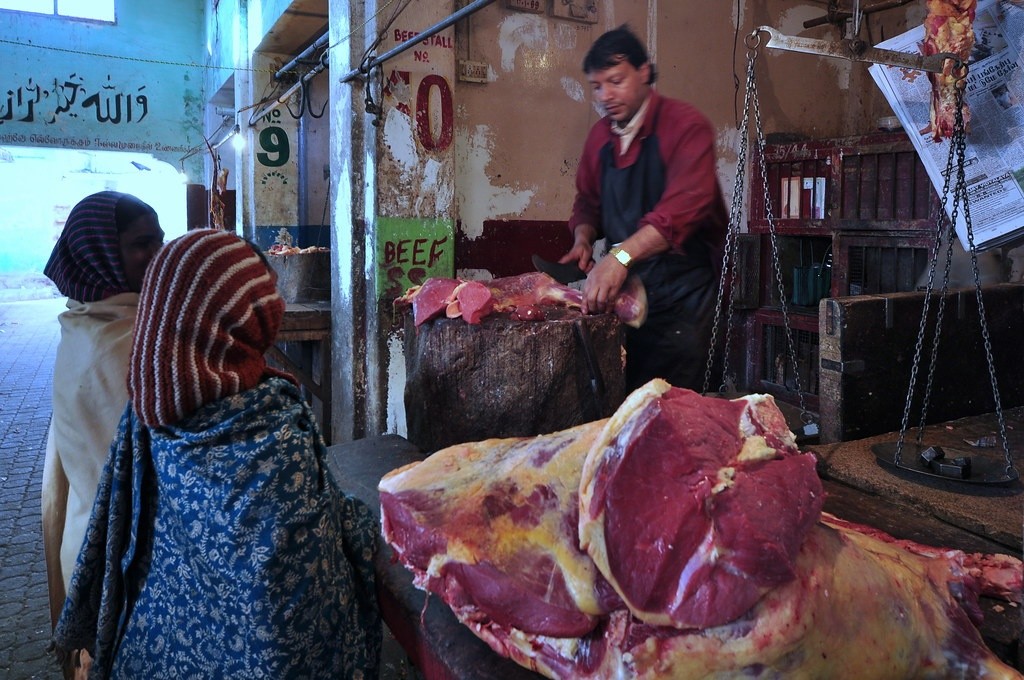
[532,254,596,284]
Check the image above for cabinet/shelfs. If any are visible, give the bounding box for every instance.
[730,131,949,417]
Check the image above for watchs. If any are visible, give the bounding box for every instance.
[609,247,635,270]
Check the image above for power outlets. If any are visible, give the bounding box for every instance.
[459,59,488,84]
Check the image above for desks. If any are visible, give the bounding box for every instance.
[272,300,333,448]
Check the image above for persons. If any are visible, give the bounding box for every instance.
[48,227,382,680]
[557,20,738,397]
[42,191,165,680]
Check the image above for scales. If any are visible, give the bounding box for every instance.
[696,1,1020,486]
